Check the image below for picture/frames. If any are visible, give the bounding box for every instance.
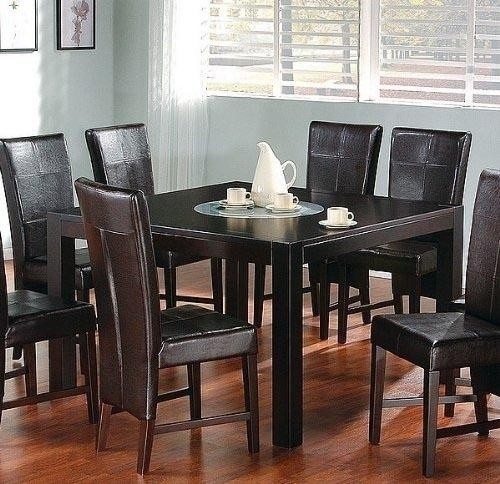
[0,0,96,52]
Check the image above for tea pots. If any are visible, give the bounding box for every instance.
[250,142,298,207]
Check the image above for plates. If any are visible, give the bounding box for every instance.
[318,220,357,229]
[220,199,255,209]
[265,204,303,213]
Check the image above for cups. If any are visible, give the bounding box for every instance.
[226,187,252,204]
[273,193,300,208]
[326,206,354,224]
